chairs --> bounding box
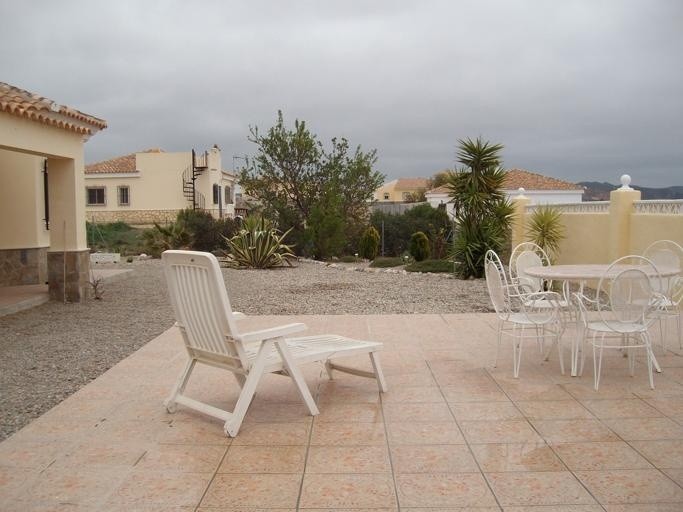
[481,240,682,393]
[157,249,386,441]
